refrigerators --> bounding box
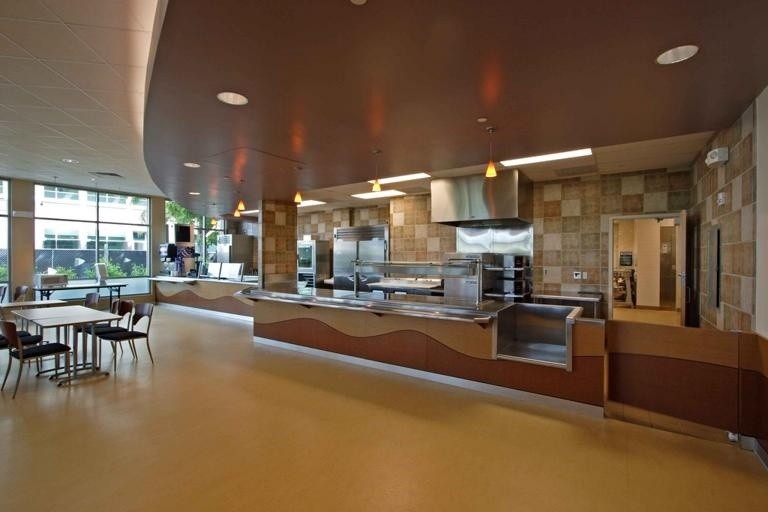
[333,227,389,292]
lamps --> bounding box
[238,180,245,211]
[234,191,240,217]
[485,126,497,178]
[293,167,303,202]
[211,203,216,224]
[372,150,382,192]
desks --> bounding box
[31,283,128,312]
[531,291,602,318]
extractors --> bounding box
[430,169,534,227]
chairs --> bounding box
[0,286,153,399]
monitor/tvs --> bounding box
[175,224,191,242]
[619,250,633,266]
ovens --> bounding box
[296,239,331,289]
